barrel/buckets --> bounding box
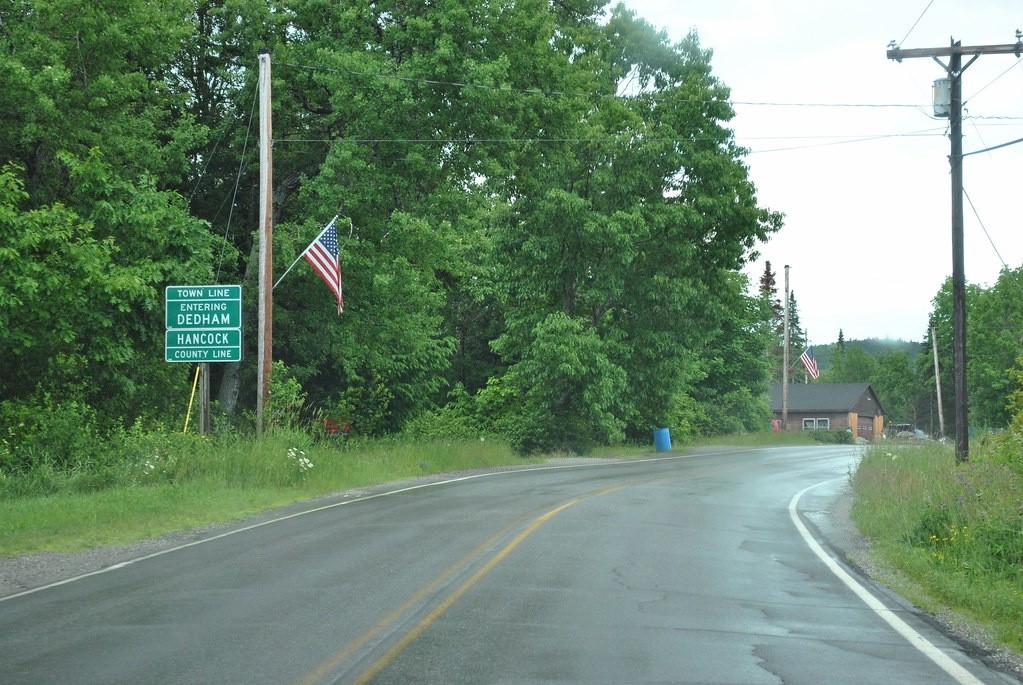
[653,427,671,452]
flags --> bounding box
[303,221,343,315]
[800,346,820,379]
